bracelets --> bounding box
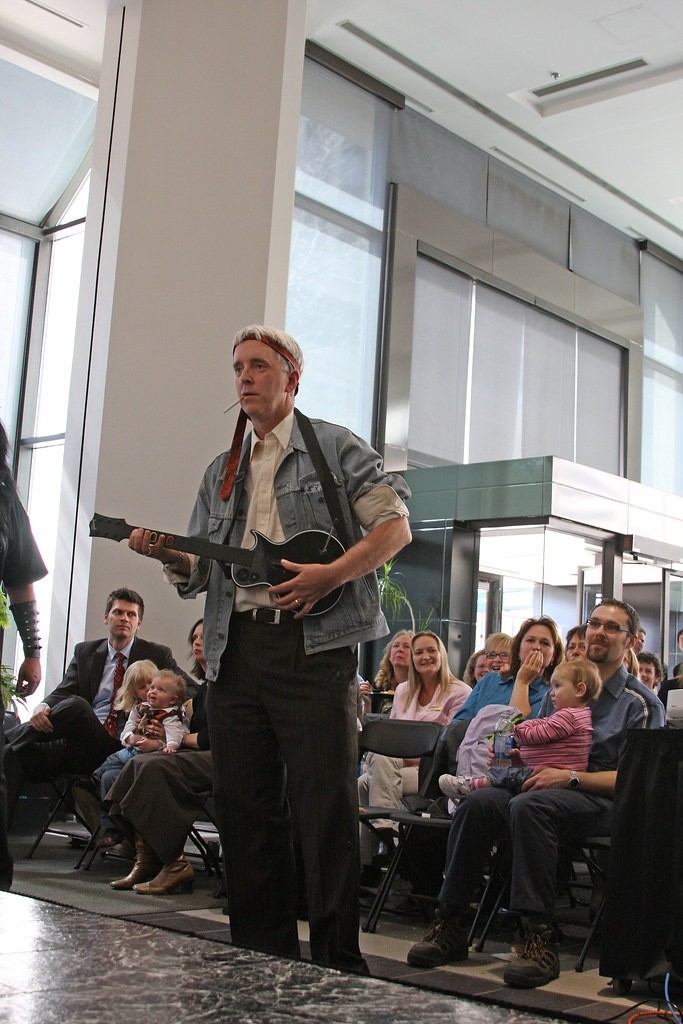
[569,771,580,789]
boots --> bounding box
[109,830,161,889]
[133,854,194,894]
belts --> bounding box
[231,608,301,625]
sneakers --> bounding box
[407,908,468,965]
[503,921,561,988]
[439,774,474,799]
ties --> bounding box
[102,651,127,737]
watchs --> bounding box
[162,551,184,570]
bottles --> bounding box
[492,710,514,767]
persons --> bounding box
[451,615,683,721]
[0,423,49,892]
[73,618,213,894]
[3,588,201,832]
[407,598,666,988]
[357,630,472,888]
[438,659,602,798]
[129,324,413,976]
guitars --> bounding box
[87,512,350,616]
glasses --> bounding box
[586,620,631,634]
[485,652,509,659]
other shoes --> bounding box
[359,865,383,887]
[111,837,130,857]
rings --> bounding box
[295,599,300,606]
[145,547,151,556]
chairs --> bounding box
[357,717,442,930]
[368,718,542,946]
[473,831,616,971]
[21,772,229,899]
